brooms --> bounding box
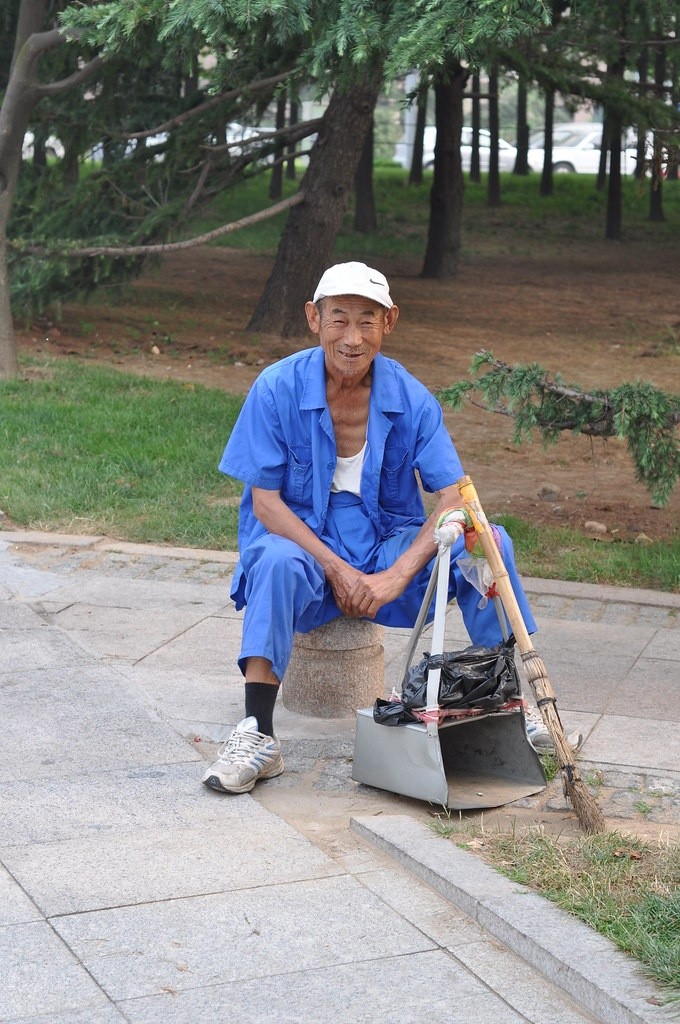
[457,474,607,837]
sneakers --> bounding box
[525,711,583,756]
[202,716,285,794]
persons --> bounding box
[202,261,583,797]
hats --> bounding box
[313,261,394,310]
